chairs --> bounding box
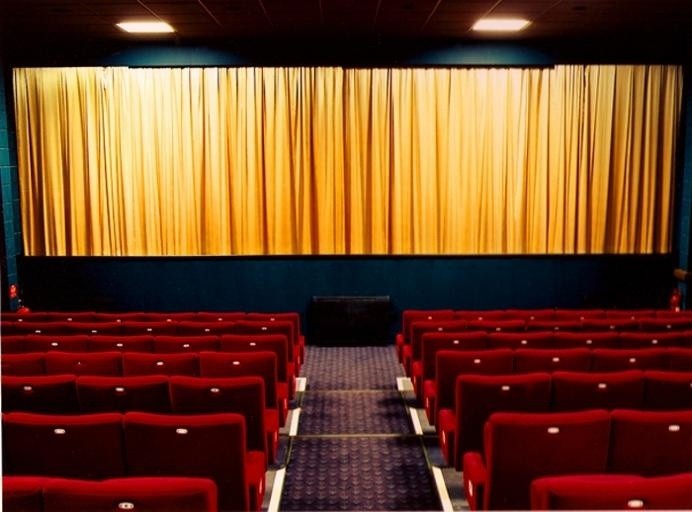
[393,303,692,510]
[0,308,313,512]
